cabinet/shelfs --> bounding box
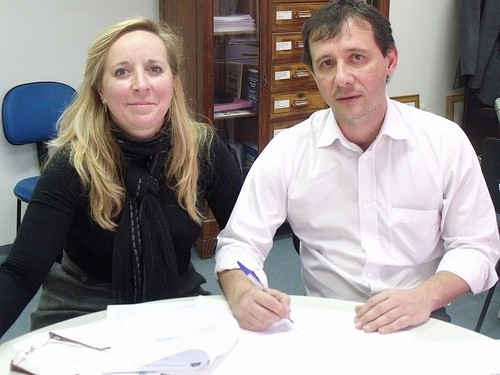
[159,0,391,259]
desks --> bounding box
[0,294,500,375]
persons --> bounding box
[214,0,500,333]
[0,17,243,337]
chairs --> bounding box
[2,81,79,231]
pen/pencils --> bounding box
[236,260,295,324]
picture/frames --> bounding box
[446,94,464,127]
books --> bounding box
[215,62,258,116]
[227,140,258,179]
